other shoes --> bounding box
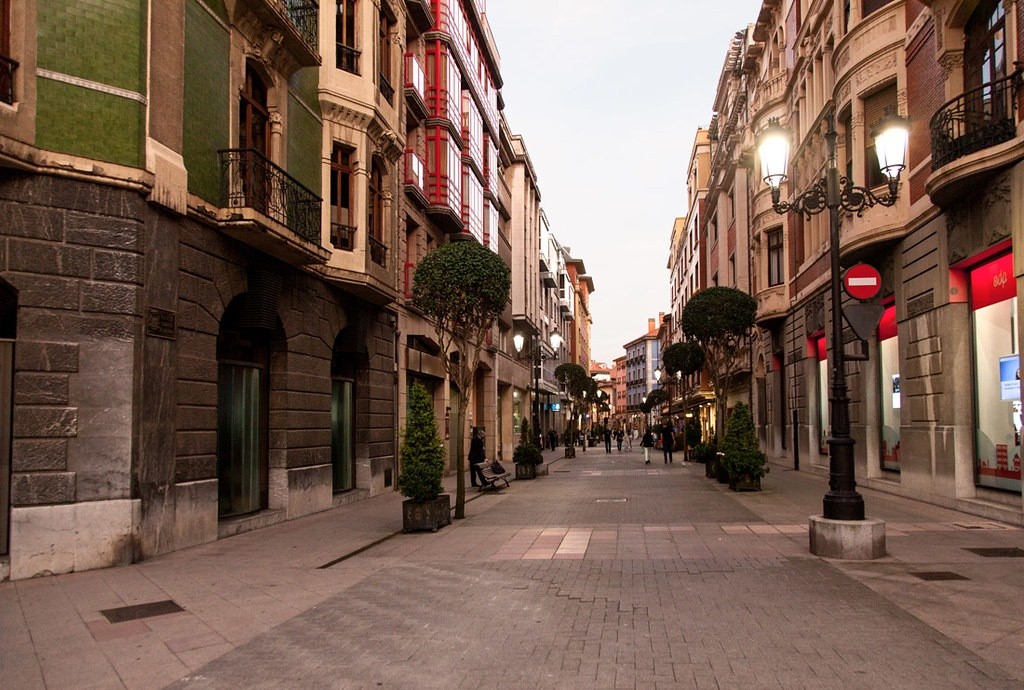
[484,482,490,487]
[472,483,480,487]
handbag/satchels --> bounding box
[491,460,505,475]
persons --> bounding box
[547,426,634,454]
[640,421,675,465]
[467,430,490,487]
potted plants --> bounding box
[720,399,766,490]
[703,428,720,477]
[399,378,450,531]
[685,415,702,462]
[513,415,543,480]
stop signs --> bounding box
[843,264,882,302]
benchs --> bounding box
[474,460,512,492]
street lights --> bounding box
[757,109,913,559]
[514,327,562,450]
[654,365,682,422]
